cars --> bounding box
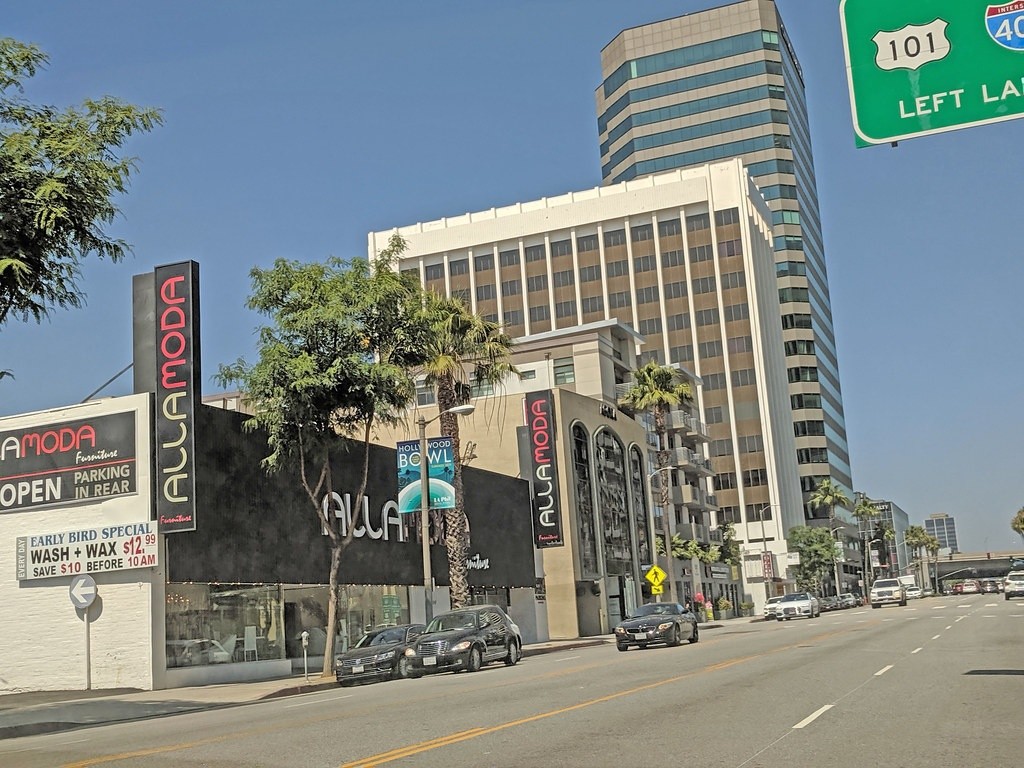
[404,604,521,676]
[335,623,428,685]
[945,564,1024,601]
[764,590,865,623]
[904,586,924,600]
[614,601,698,652]
[923,588,935,597]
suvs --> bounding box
[869,578,908,609]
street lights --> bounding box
[417,402,478,628]
[646,465,679,603]
[830,524,846,596]
[759,504,781,598]
[867,539,883,583]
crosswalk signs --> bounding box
[645,565,669,587]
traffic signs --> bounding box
[836,0,1024,158]
